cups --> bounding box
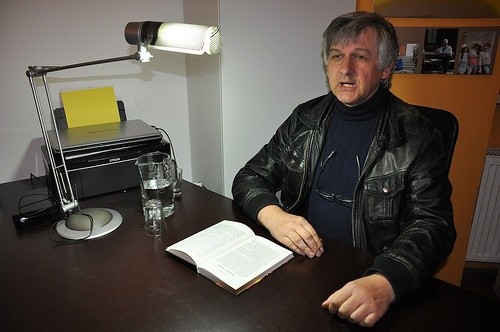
[173,168,182,198]
[143,198,168,236]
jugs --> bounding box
[135,151,176,219]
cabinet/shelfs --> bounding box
[355,0,500,287]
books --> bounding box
[164,219,293,296]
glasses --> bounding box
[316,151,361,207]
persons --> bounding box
[231,10,460,326]
[435,39,454,74]
[457,39,492,74]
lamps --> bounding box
[26,21,222,239]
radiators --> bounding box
[464,151,500,263]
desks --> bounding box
[1,173,500,332]
